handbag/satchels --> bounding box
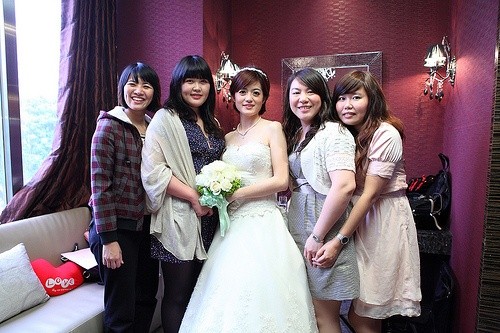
[60,242,100,284]
[404,192,443,231]
[426,152,451,230]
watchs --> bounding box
[337,233,349,244]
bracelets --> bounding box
[311,234,324,243]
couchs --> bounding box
[0,207,105,333]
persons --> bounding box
[178,67,319,333]
[89,62,163,333]
[141,55,225,333]
[312,70,423,333]
[280,68,360,333]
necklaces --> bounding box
[237,117,261,136]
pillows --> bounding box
[31,257,85,296]
[0,242,51,322]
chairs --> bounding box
[406,152,450,230]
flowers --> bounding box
[195,160,241,197]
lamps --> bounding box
[423,32,456,102]
[215,51,240,110]
